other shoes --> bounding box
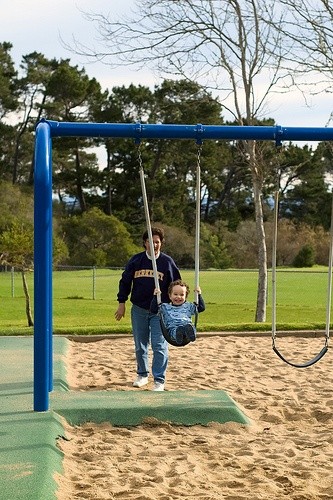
[176,325,183,344]
[132,375,148,388]
[183,323,195,342]
[150,381,164,392]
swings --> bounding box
[271,148,333,368]
[137,146,203,347]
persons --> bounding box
[150,279,205,347]
[115,228,182,391]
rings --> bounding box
[116,314,117,315]
